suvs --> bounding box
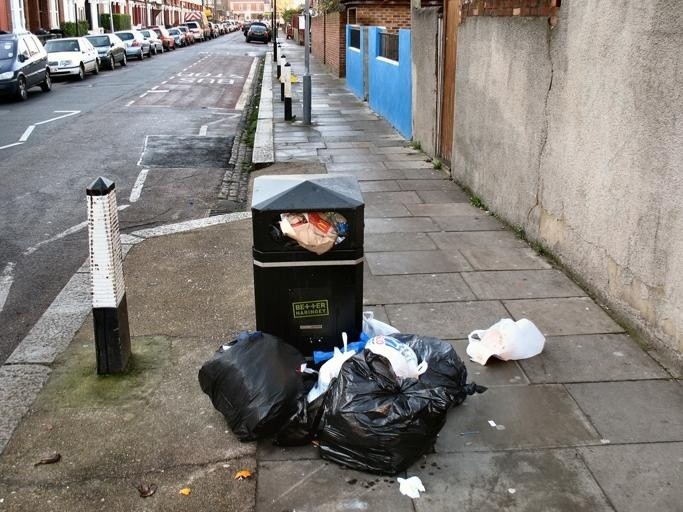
[0,31,52,101]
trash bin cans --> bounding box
[250,172,366,362]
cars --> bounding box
[83,33,128,70]
[113,19,271,71]
[42,36,101,81]
